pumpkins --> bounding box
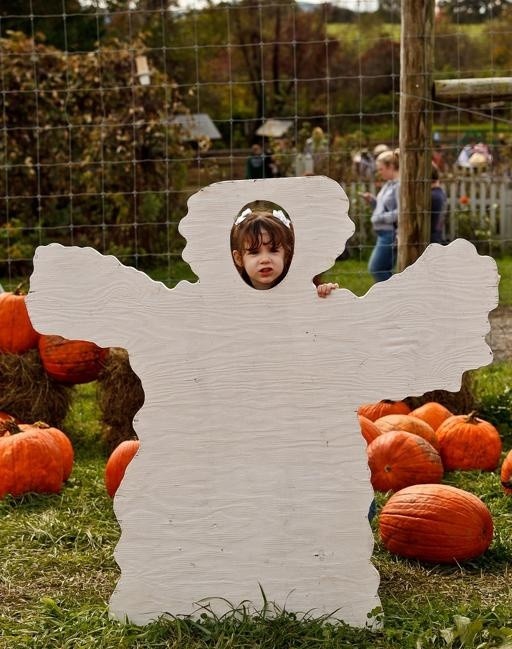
[358,401,512,563]
[0,279,139,503]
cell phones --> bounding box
[357,192,364,197]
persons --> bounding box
[357,150,401,288]
[429,162,448,246]
[247,139,316,178]
[229,206,344,300]
